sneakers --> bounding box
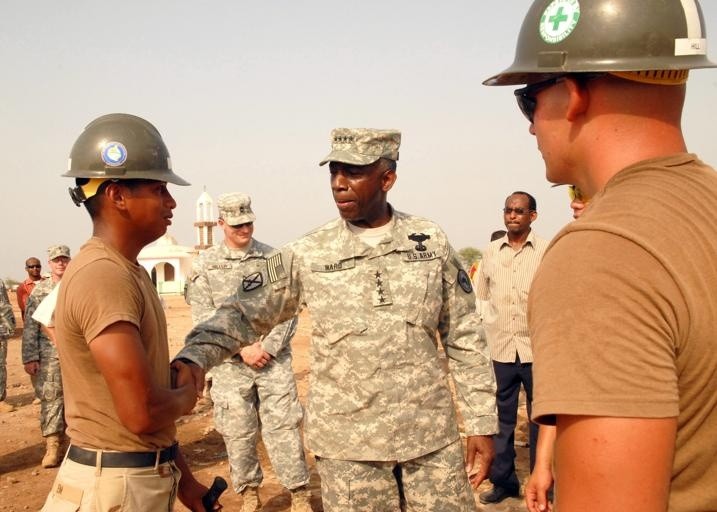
[479,486,519,504]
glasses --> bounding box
[504,207,531,214]
[514,73,590,124]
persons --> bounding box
[477,0,717,512]
[181,193,313,512]
[0,278,18,403]
[38,112,229,512]
[476,191,557,504]
[21,244,72,470]
[169,123,500,512]
[522,181,589,512]
[18,257,48,407]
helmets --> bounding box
[481,0,717,85]
[59,114,190,186]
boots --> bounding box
[42,432,70,468]
[290,487,312,512]
[239,488,263,512]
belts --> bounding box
[67,440,178,467]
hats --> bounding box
[48,245,70,260]
[219,193,255,226]
[319,127,400,166]
